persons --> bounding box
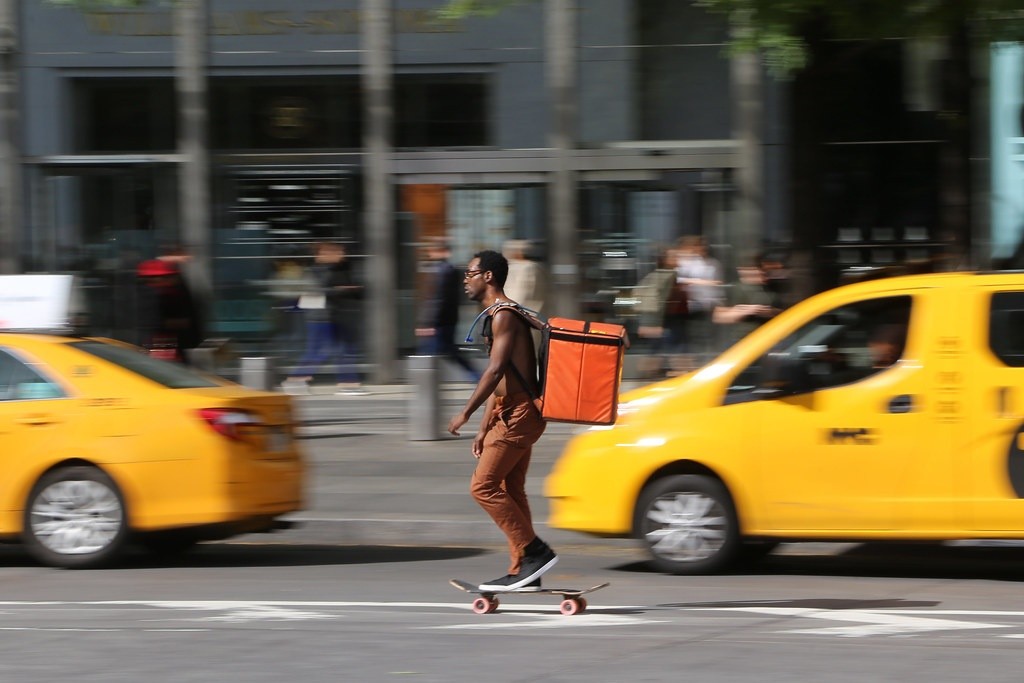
[449,250,560,596]
[255,232,904,395]
[133,242,193,365]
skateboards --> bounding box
[448,577,611,616]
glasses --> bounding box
[463,269,487,278]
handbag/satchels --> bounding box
[297,292,326,309]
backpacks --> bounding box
[481,306,631,426]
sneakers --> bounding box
[479,574,541,594]
[507,536,560,589]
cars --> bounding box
[0,334,308,569]
[542,270,1023,576]
